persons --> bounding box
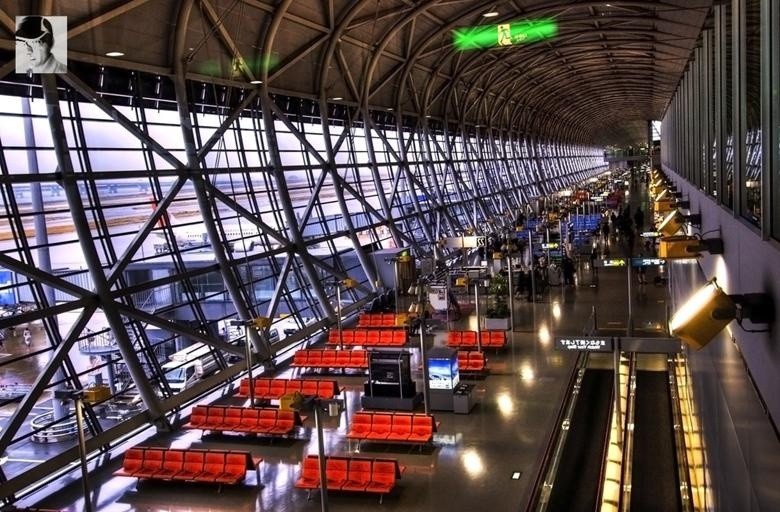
[15,16,67,73]
[23,327,31,348]
[635,253,647,283]
[515,248,598,299]
[603,204,644,242]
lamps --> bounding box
[644,160,725,263]
[667,277,770,353]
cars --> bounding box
[283,318,316,336]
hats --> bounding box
[15,17,52,42]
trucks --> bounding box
[159,342,230,402]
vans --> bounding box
[229,328,280,361]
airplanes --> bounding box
[139,197,291,253]
